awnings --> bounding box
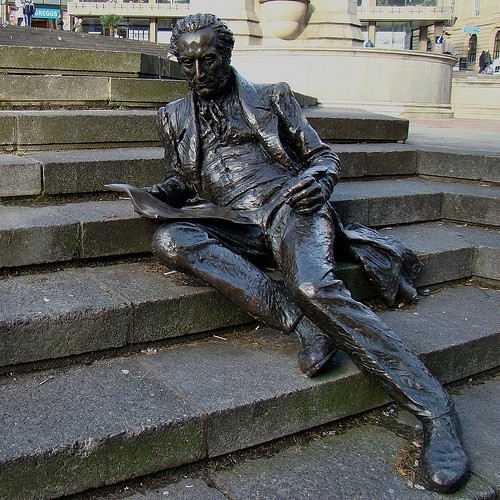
[463,27,480,34]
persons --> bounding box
[16,0,84,33]
[133,13,473,492]
[478,49,492,73]
[365,39,374,47]
